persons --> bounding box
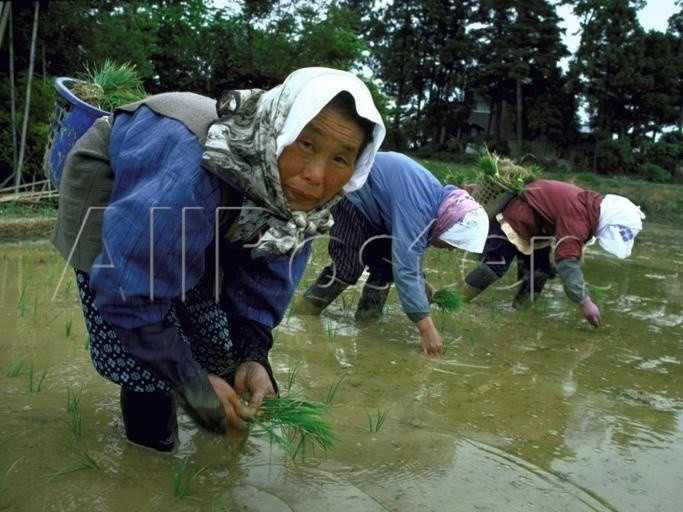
[451,179,643,328]
[288,150,490,358]
[49,67,387,454]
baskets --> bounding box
[471,172,514,217]
[42,76,111,189]
